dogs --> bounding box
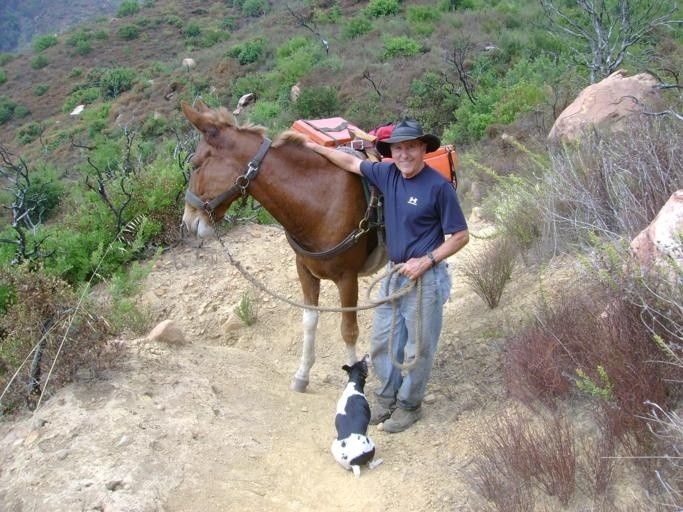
[330,353,383,479]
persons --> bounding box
[280,120,469,432]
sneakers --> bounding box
[368,405,396,425]
[377,410,421,431]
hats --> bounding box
[376,116,440,157]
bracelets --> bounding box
[425,250,435,268]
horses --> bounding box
[180,96,389,393]
